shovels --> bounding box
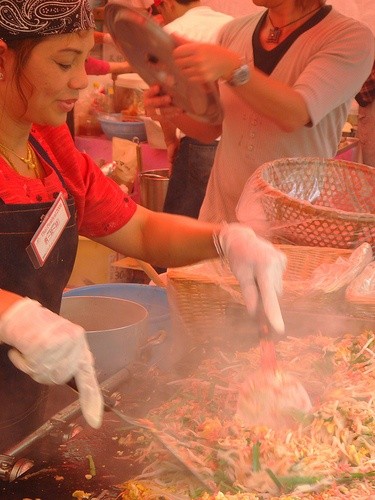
[236,278,312,430]
[65,376,219,493]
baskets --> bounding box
[167,246,375,342]
[257,159,375,247]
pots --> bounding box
[58,296,166,377]
[137,169,170,212]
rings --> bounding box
[155,108,161,115]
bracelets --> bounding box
[227,63,250,85]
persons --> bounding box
[143,0,375,224]
[354,61,375,170]
[153,0,237,274]
[0,0,287,456]
[66,30,139,141]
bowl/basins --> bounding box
[96,113,146,141]
[63,281,172,345]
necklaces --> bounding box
[266,6,321,43]
[0,144,40,181]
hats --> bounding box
[0,0,97,40]
